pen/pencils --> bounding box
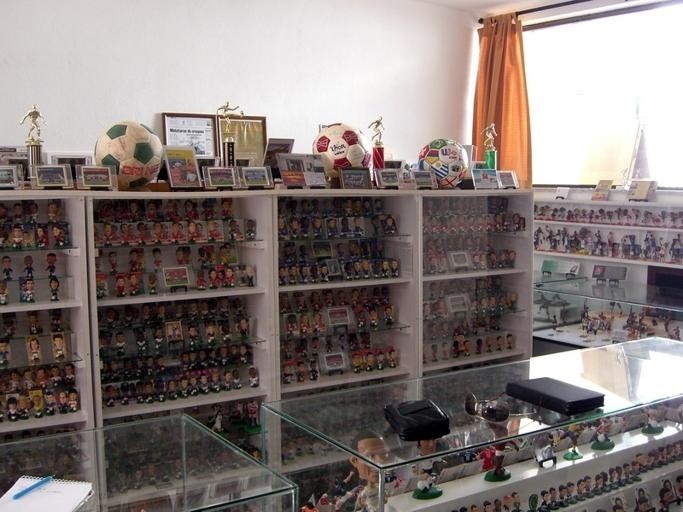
[13,474,56,500]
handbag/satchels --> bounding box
[383,398,450,442]
[506,377,605,415]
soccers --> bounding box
[94,121,163,190]
[418,139,469,189]
[312,122,371,179]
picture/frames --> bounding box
[163,112,267,160]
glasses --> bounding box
[463,392,543,424]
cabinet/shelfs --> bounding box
[0,189,535,512]
[380,396,683,512]
[535,199,682,352]
